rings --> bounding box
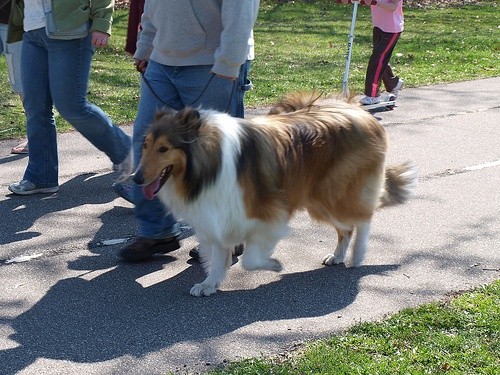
[102,39,106,41]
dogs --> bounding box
[132,87,416,297]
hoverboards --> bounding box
[336,0,397,112]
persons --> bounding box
[335,0,404,105]
[0,0,260,259]
[117,0,261,259]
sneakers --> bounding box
[190,244,244,257]
[8,179,59,195]
[359,96,381,104]
[391,79,404,96]
[119,237,180,259]
[111,150,132,187]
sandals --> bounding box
[11,142,28,154]
